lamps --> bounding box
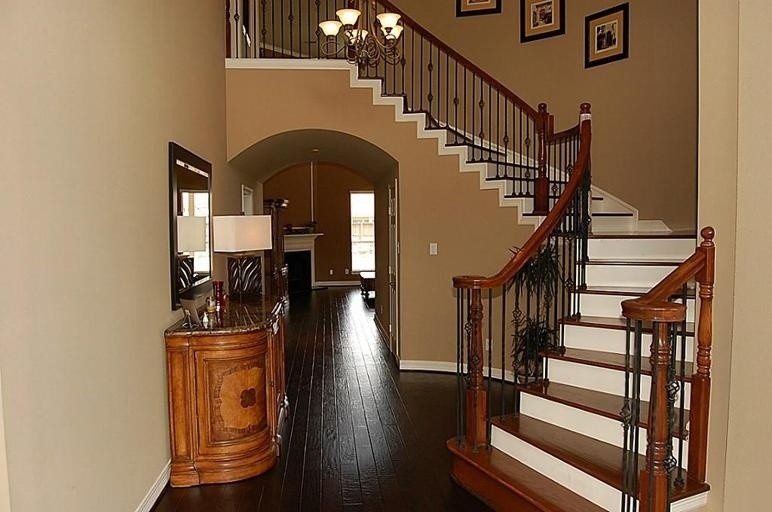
[176,215,206,252]
[315,0,405,69]
[211,213,274,307]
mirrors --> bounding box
[168,141,213,311]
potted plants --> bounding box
[502,242,567,389]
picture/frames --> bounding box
[519,0,567,45]
[582,1,631,72]
[454,0,504,19]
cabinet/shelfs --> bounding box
[163,297,291,489]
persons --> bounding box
[533,2,551,27]
[597,29,616,49]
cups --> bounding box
[206,300,217,313]
